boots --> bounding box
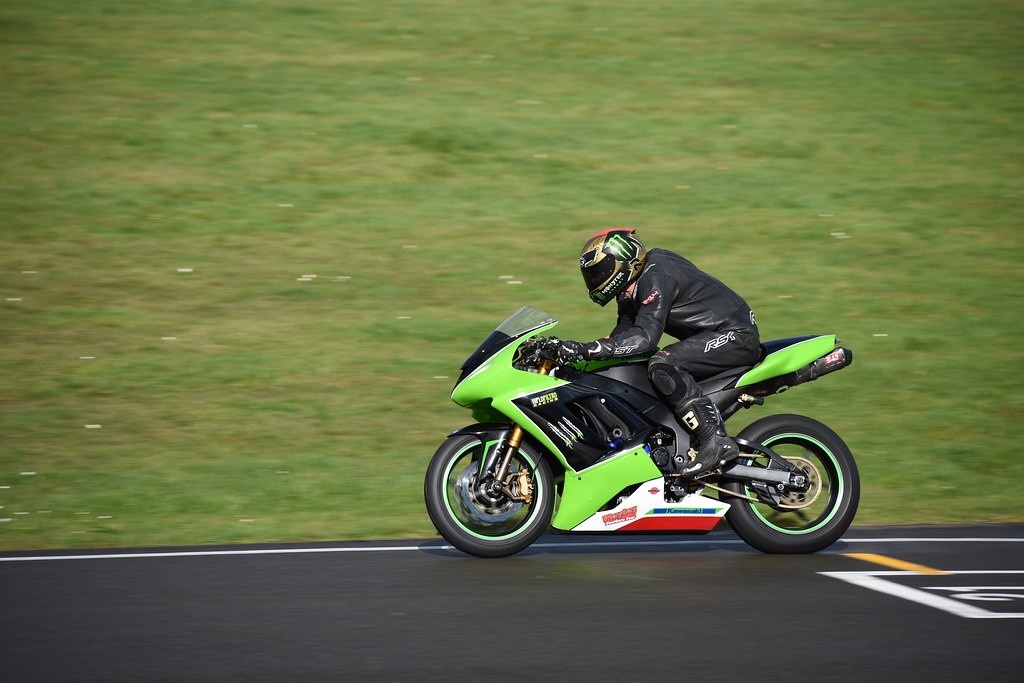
[678,395,740,477]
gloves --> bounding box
[558,337,591,364]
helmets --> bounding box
[580,227,646,307]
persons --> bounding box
[521,228,760,477]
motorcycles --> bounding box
[424,305,861,559]
